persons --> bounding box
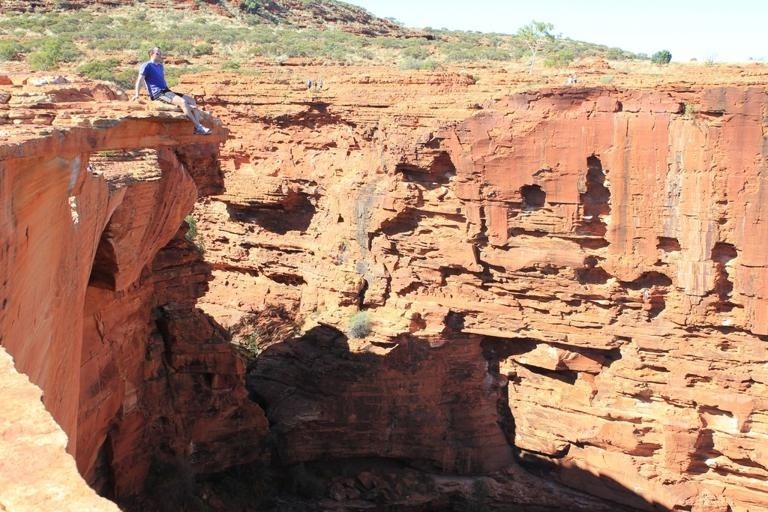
[133,47,212,134]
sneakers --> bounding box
[193,124,211,134]
